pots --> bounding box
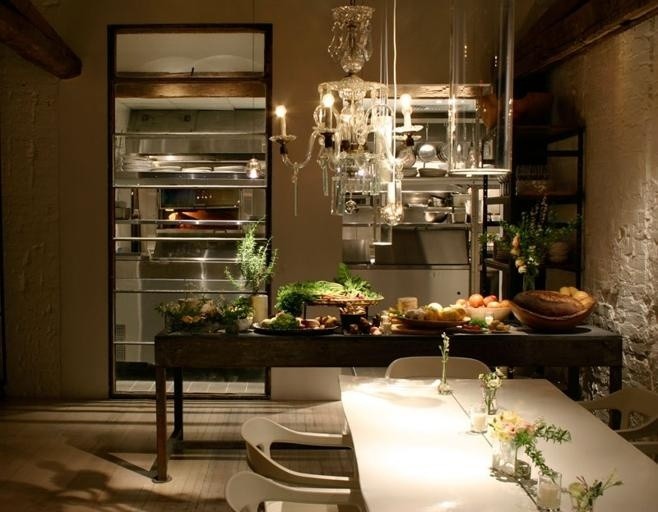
[415,125,448,163]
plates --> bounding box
[395,316,471,327]
[123,155,245,174]
[253,323,339,334]
[403,168,447,177]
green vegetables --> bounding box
[275,279,347,316]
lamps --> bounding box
[267,0,425,227]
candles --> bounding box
[538,472,562,508]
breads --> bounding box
[514,286,594,316]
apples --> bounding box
[457,294,512,309]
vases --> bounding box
[478,391,500,415]
[490,438,517,476]
[523,274,535,289]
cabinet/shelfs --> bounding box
[480,122,585,320]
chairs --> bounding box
[576,382,658,440]
[627,441,657,463]
[239,417,351,489]
[384,356,496,379]
[225,470,351,512]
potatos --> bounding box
[261,312,338,328]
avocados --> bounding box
[347,316,370,334]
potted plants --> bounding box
[221,213,280,332]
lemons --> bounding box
[426,302,466,321]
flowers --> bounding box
[487,410,534,440]
[474,367,509,389]
[569,467,619,511]
[477,202,582,290]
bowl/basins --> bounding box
[423,210,448,223]
[451,302,597,333]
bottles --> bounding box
[246,158,261,178]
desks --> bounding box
[154,322,622,483]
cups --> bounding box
[382,317,392,336]
[484,312,495,325]
[537,469,561,512]
[471,405,488,433]
